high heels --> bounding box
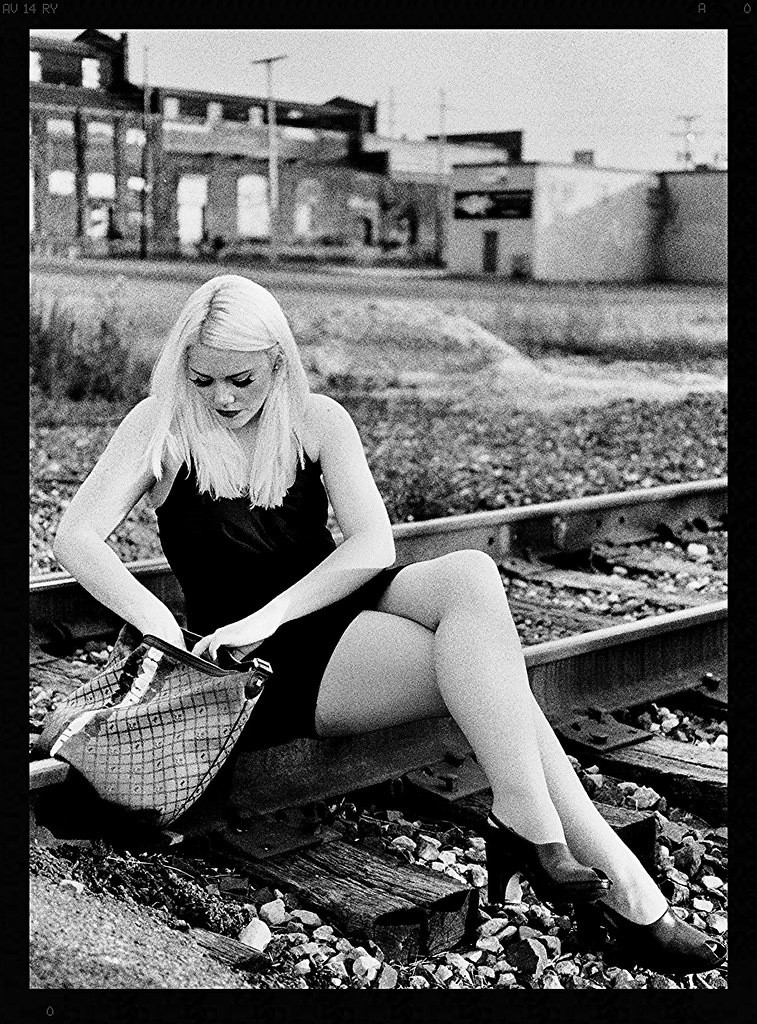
[480,808,614,908]
[574,892,729,975]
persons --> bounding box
[51,275,726,974]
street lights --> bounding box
[252,52,289,267]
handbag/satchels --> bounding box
[31,610,276,841]
